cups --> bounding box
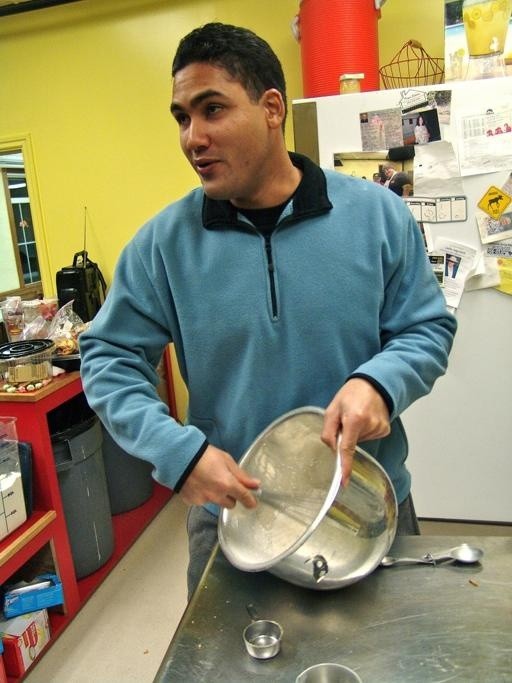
[242,602,283,660]
[377,546,485,569]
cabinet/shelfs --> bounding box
[0,343,179,683]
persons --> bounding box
[78,20,459,608]
[414,114,431,146]
[446,255,460,279]
[487,215,512,235]
[382,161,412,197]
[372,172,385,186]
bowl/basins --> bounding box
[217,405,399,591]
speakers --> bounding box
[56,262,101,323]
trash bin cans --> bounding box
[50,408,156,578]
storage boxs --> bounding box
[0,606,50,678]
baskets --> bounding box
[379,40,444,89]
